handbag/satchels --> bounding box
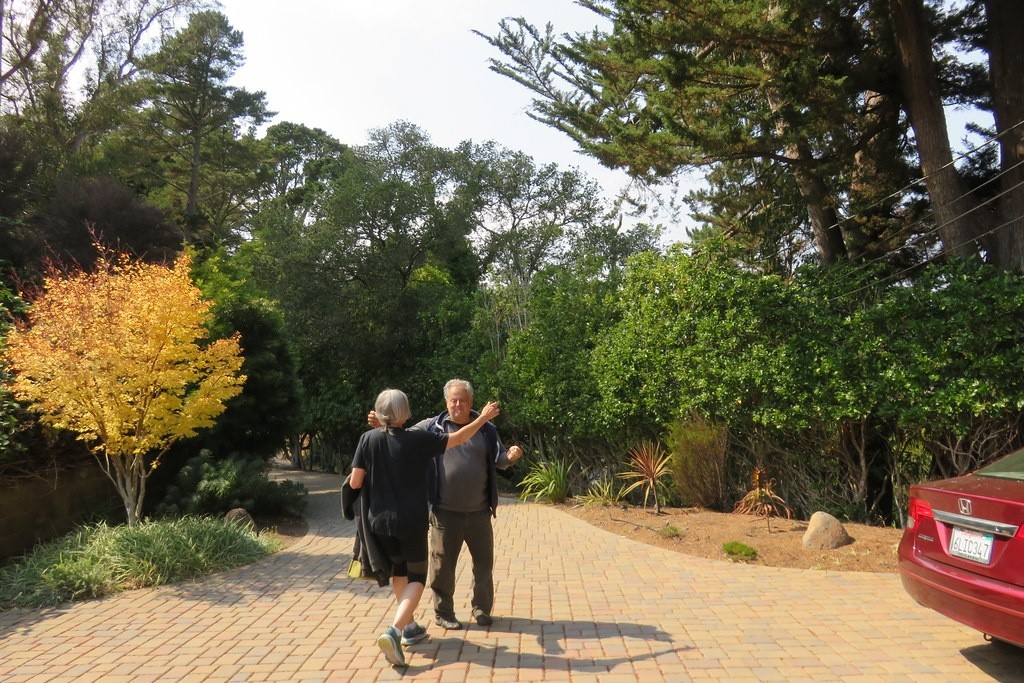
[347,559,376,582]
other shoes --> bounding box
[474,609,492,625]
[436,616,463,629]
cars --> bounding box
[898,446,1024,655]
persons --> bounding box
[346,388,500,668]
[368,378,523,631]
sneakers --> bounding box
[400,622,427,645]
[378,624,405,666]
